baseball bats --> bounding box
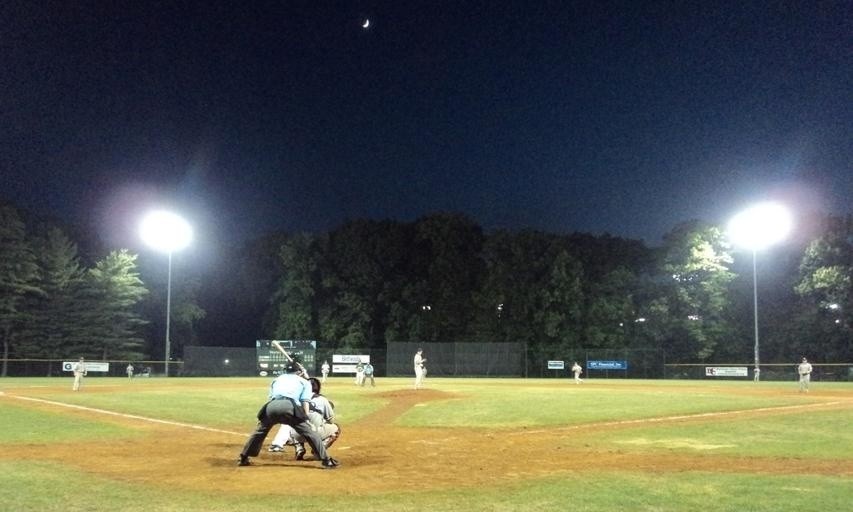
[272,340,293,363]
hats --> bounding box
[284,362,301,372]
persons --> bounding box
[413,348,427,389]
[753,366,760,380]
[571,362,582,384]
[356,361,365,384]
[798,357,813,393]
[72,357,87,390]
[127,363,134,380]
[362,362,374,386]
[237,356,340,467]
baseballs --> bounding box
[225,359,230,363]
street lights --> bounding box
[136,210,192,373]
[734,202,790,380]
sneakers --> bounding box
[294,443,306,461]
[268,445,285,453]
[322,457,342,468]
[237,453,250,467]
